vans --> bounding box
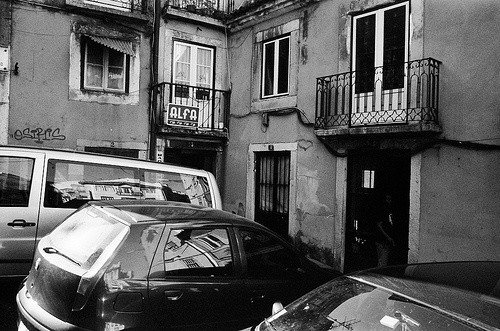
[0,144,224,279]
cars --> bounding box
[15,198,344,331]
[237,259,500,331]
